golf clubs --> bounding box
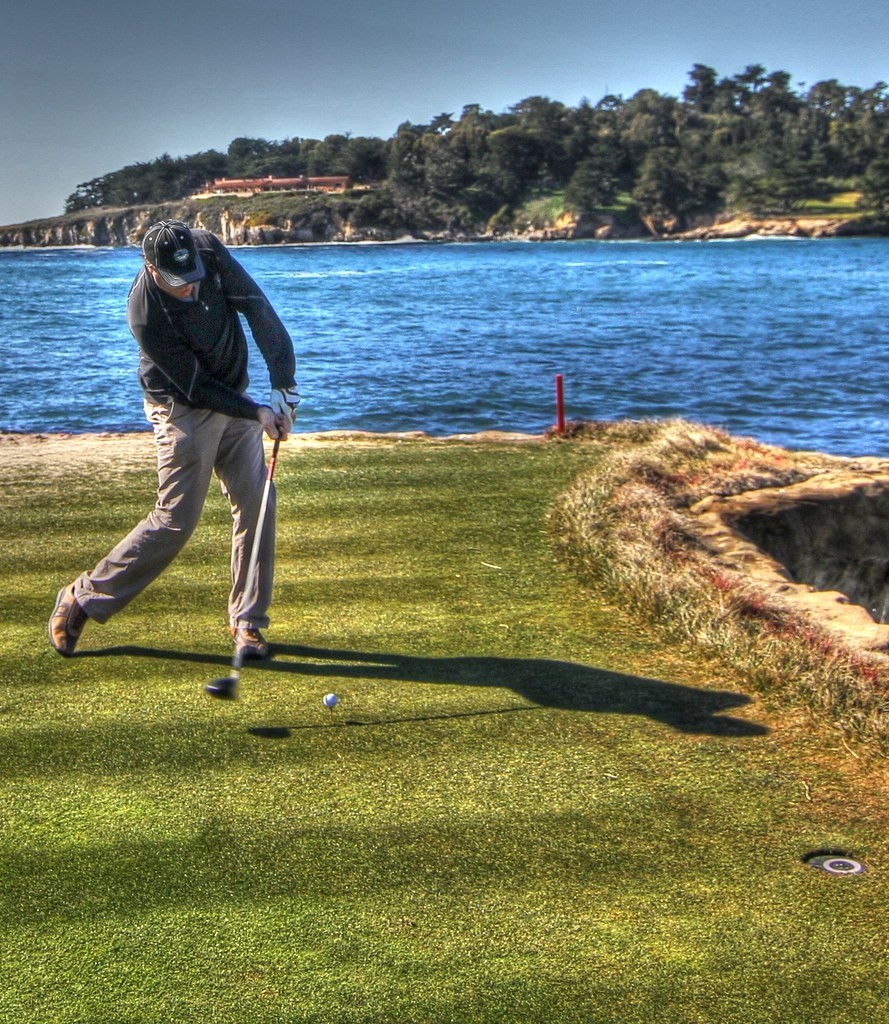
[205,397,286,702]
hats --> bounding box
[142,219,204,288]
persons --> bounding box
[48,220,299,659]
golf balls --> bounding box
[319,690,341,710]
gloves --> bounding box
[271,388,300,424]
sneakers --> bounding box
[229,626,268,661]
[48,580,85,659]
